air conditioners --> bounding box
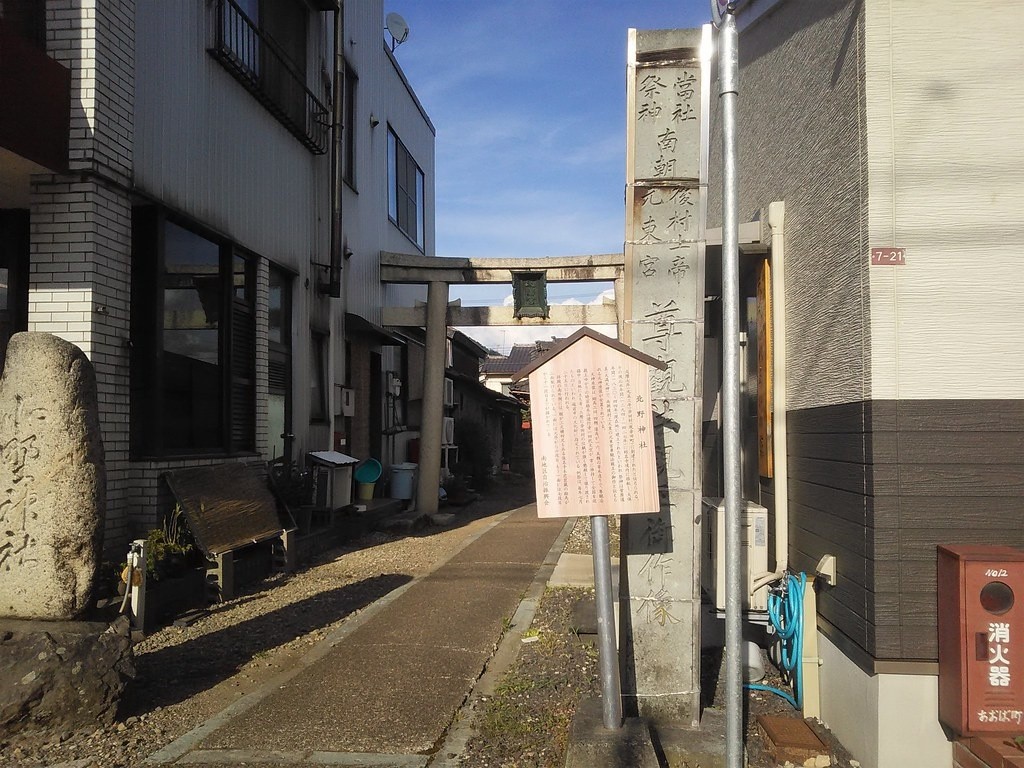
[701,497,768,614]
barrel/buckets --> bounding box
[389,469,417,499]
[357,482,376,501]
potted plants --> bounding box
[271,461,319,533]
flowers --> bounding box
[95,529,185,597]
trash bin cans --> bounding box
[388,462,416,500]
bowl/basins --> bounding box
[353,457,382,483]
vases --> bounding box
[98,566,204,618]
